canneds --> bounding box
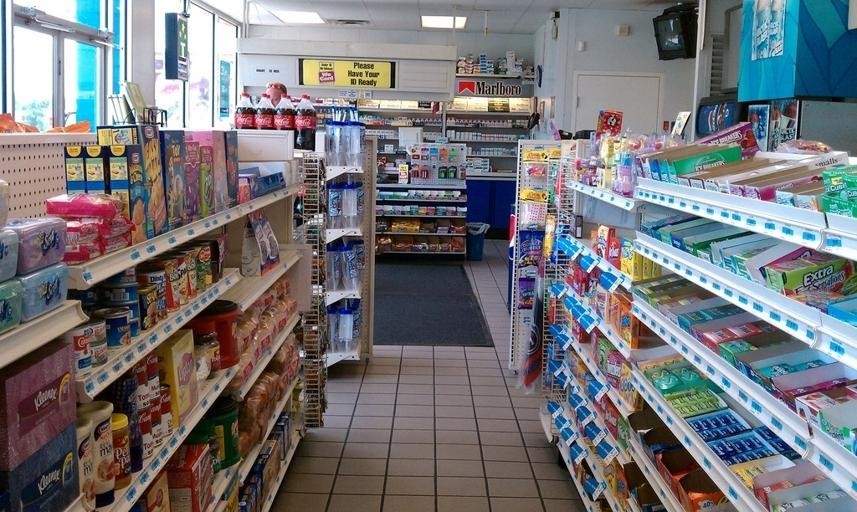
[59,240,221,493]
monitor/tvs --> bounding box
[718,94,769,132]
[694,96,723,138]
[652,2,699,61]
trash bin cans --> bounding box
[466,222,484,261]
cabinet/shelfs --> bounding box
[375,141,473,260]
[506,135,565,397]
[358,98,538,184]
[0,112,332,512]
[537,133,857,512]
[735,0,857,105]
[466,176,519,240]
[320,128,378,377]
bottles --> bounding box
[359,114,442,127]
[321,120,367,356]
[234,92,315,152]
[446,116,531,156]
